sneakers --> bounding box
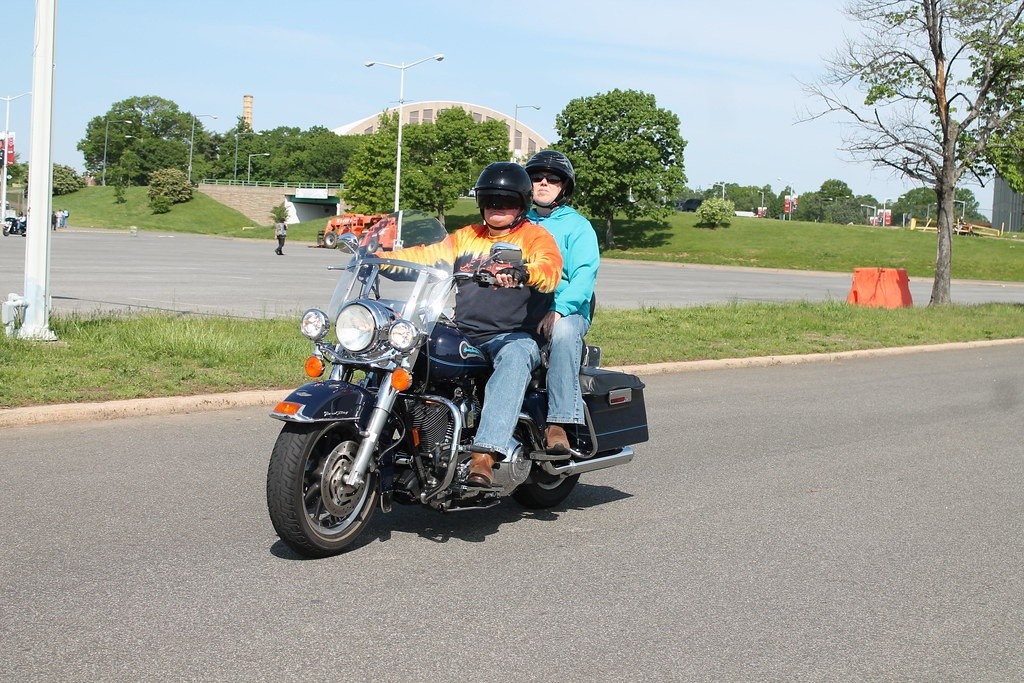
[544,425,570,454]
[466,452,495,488]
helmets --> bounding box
[475,162,533,208]
[526,150,575,197]
[18,211,23,216]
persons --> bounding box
[523,151,600,455]
[16,211,26,233]
[351,162,564,490]
[51,208,70,231]
[275,218,288,255]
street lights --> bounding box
[234,132,265,182]
[247,152,273,185]
[364,55,447,214]
[187,114,225,186]
[777,178,794,222]
[1,92,34,228]
[882,195,906,228]
[758,190,764,218]
[101,118,137,188]
[714,183,726,202]
[860,204,876,227]
[512,104,543,123]
[953,199,968,221]
[927,202,937,221]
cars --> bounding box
[671,197,702,212]
[364,213,448,246]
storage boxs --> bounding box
[580,366,649,452]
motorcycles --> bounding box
[2,216,28,237]
[263,209,638,553]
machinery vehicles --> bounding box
[314,211,392,251]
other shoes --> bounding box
[275,249,279,255]
[278,253,284,255]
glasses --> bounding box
[530,172,562,184]
[477,189,522,208]
